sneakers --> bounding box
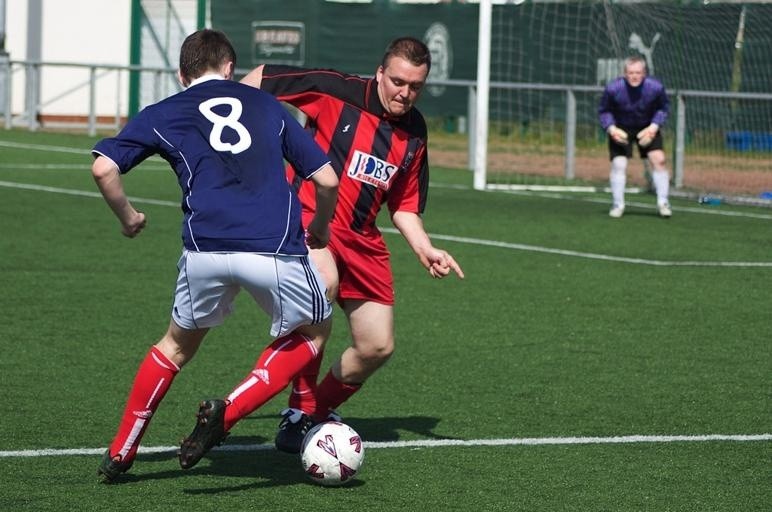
[658,203,672,217]
[97,449,134,484]
[275,407,342,455]
[176,399,231,469]
[609,205,625,218]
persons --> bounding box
[237,37,465,453]
[91,29,339,485]
[597,52,672,219]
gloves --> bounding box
[607,124,629,146]
[637,122,659,147]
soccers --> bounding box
[299,421,364,488]
